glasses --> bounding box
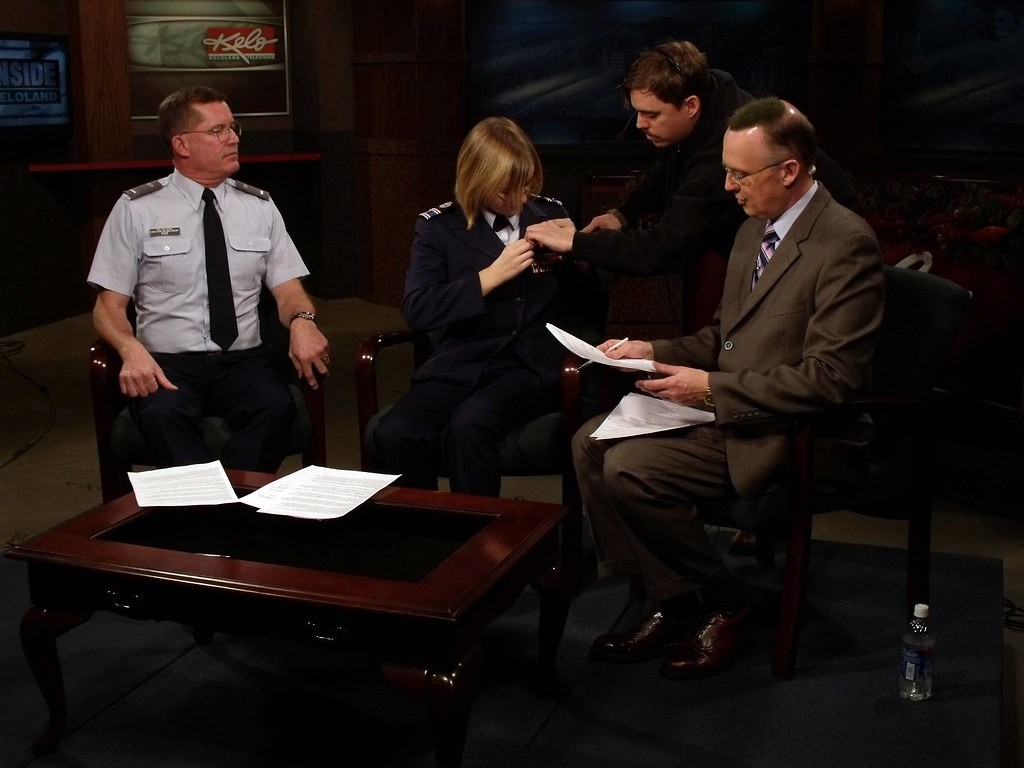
[177,120,243,144]
[495,171,542,205]
[719,155,793,182]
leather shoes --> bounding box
[665,585,756,673]
[590,562,697,680]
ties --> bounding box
[751,224,781,291]
[201,188,239,350]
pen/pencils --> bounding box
[575,337,629,372]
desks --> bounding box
[0,468,570,768]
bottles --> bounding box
[897,604,937,702]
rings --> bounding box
[321,352,329,361]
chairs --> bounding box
[87,282,328,503]
[628,261,974,683]
[354,257,611,599]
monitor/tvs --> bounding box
[0,32,74,140]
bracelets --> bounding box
[607,208,628,228]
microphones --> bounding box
[616,111,637,143]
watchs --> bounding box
[289,311,318,326]
[703,385,715,413]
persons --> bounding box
[87,85,332,498]
[571,98,885,672]
[526,40,864,277]
[373,117,576,499]
